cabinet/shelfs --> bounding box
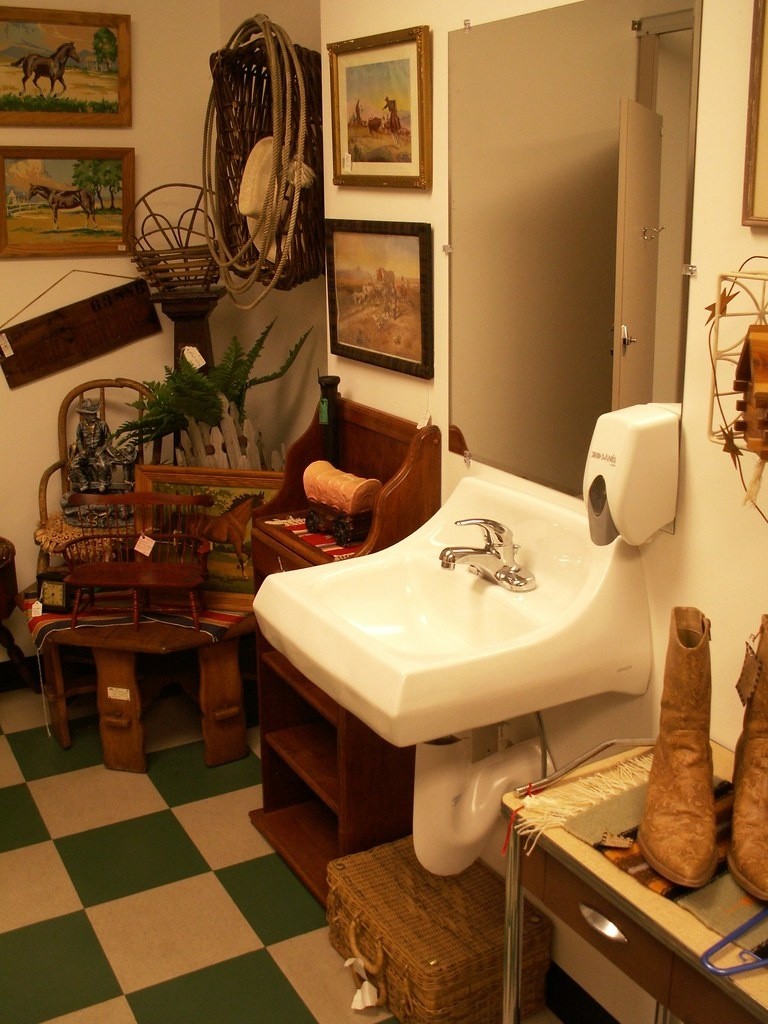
[247,393,444,909]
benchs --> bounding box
[53,532,212,631]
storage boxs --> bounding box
[40,581,68,614]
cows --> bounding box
[368,116,386,137]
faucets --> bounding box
[438,518,537,591]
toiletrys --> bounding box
[583,401,679,546]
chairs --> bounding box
[64,485,217,668]
[33,376,164,585]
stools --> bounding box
[0,536,42,704]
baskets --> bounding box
[125,184,224,296]
[210,35,326,290]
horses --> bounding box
[11,42,80,96]
[26,183,98,232]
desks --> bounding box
[22,590,256,776]
[501,737,768,1024]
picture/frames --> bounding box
[0,5,132,126]
[322,217,436,381]
[132,460,287,611]
[737,0,768,229]
[325,23,434,193]
[0,144,138,260]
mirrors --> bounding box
[446,0,704,537]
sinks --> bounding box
[250,467,653,747]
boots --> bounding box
[727,614,768,902]
[637,607,718,888]
